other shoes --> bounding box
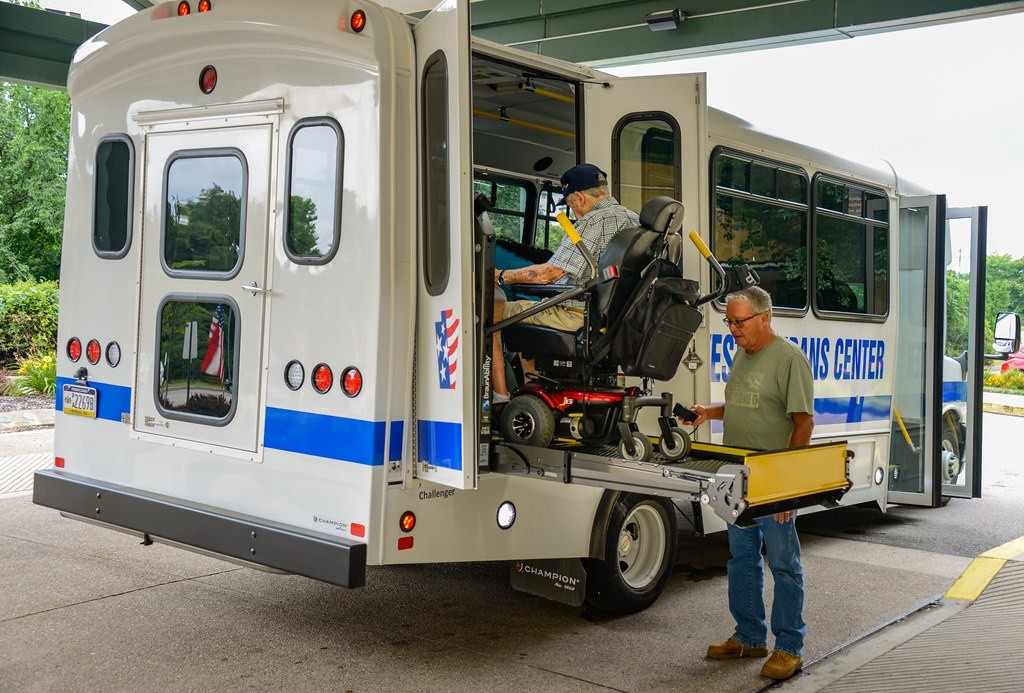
[490,402,512,435]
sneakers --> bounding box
[761,650,804,679]
[707,635,768,660]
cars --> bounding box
[1001,344,1024,374]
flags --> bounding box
[200,306,224,387]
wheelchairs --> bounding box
[491,190,729,463]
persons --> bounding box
[491,164,641,414]
[676,285,814,679]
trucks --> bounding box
[26,1,1024,620]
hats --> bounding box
[555,164,607,206]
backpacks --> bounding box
[609,243,703,381]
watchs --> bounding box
[498,270,505,285]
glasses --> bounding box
[723,313,763,329]
